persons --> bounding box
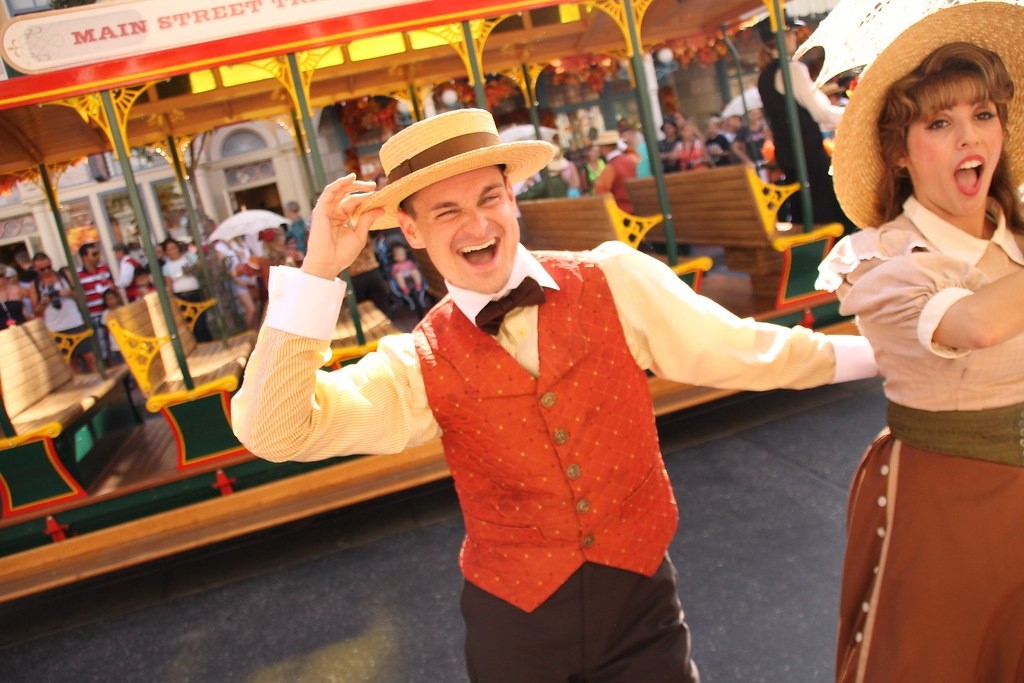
[230,106,882,683]
[0,173,429,372]
[753,9,859,238]
[814,0,1024,683]
[548,107,790,196]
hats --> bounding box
[830,3,1024,232]
[660,116,678,132]
[351,106,561,233]
[758,9,808,41]
[593,129,620,146]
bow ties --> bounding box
[473,275,547,335]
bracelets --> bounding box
[41,301,47,306]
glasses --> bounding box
[91,251,101,257]
[39,266,52,271]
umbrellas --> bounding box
[719,88,765,123]
[206,205,290,254]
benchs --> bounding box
[103,285,258,414]
[516,193,713,275]
[626,162,844,251]
[285,250,404,371]
[0,318,129,448]
